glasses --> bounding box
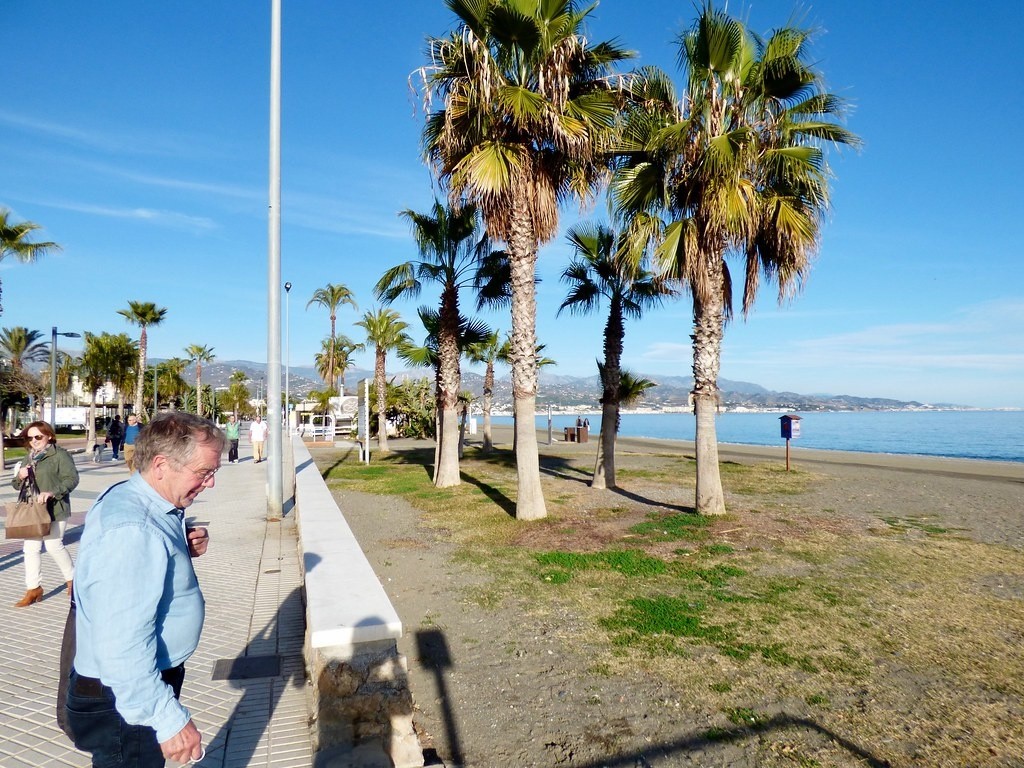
[27,435,45,441]
[176,460,219,481]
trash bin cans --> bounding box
[564,427,575,442]
[577,427,588,443]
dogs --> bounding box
[93,443,108,462]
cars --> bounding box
[242,413,267,422]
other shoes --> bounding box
[229,459,238,464]
[254,460,261,463]
[112,458,117,461]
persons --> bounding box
[225,415,240,464]
[12,420,81,608]
[247,415,268,463]
[105,413,146,476]
[56,410,234,768]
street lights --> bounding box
[51,326,82,432]
[154,364,170,418]
[285,281,292,437]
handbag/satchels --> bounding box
[56,607,76,742]
[5,466,51,539]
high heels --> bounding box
[15,586,43,607]
[66,581,73,598]
